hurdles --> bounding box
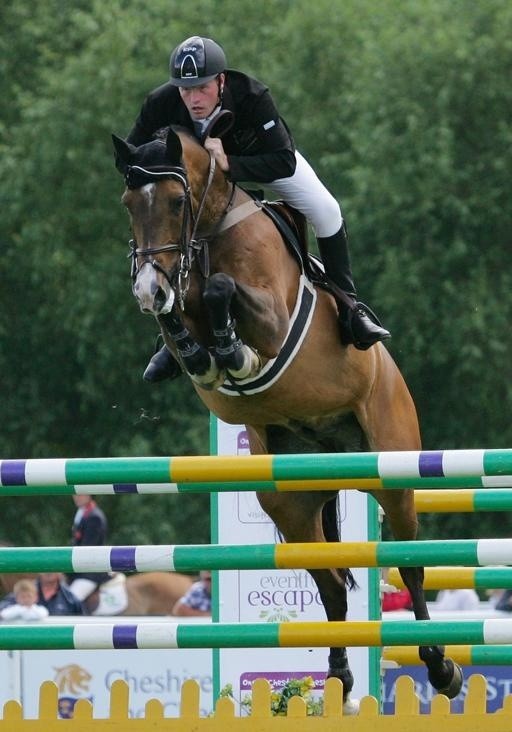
[0,445,512,730]
[209,410,512,715]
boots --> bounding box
[141,342,175,386]
[315,222,392,345]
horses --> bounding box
[0,542,202,618]
[108,125,462,704]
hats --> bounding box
[169,35,226,85]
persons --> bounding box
[481,562,512,613]
[1,572,88,616]
[2,579,50,624]
[68,492,117,610]
[127,31,396,387]
[429,586,479,614]
[174,567,212,618]
